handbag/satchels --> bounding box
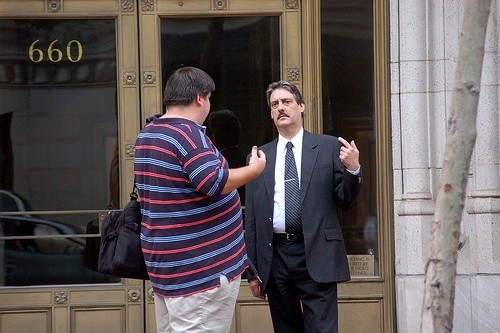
[97,190,150,281]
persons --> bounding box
[133,66,266,333]
[106,149,119,209]
[210,107,247,205]
[245,80,362,333]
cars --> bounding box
[0,214,88,284]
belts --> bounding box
[273,231,303,242]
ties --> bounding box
[283,142,303,234]
